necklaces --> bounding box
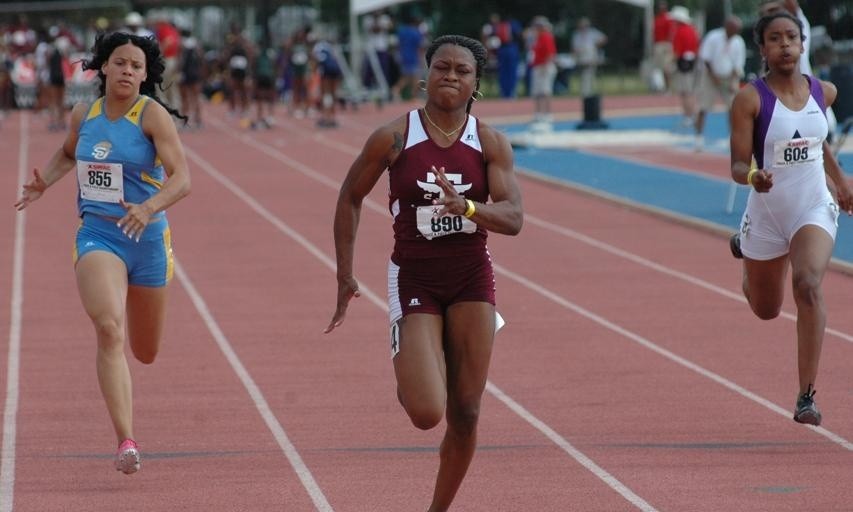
[424,107,468,143]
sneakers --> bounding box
[114,439,140,475]
[793,394,821,425]
[730,233,743,260]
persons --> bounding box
[720,4,851,426]
[11,27,194,476]
[0,0,852,138]
[320,32,528,512]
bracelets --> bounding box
[462,198,476,219]
[746,168,759,186]
